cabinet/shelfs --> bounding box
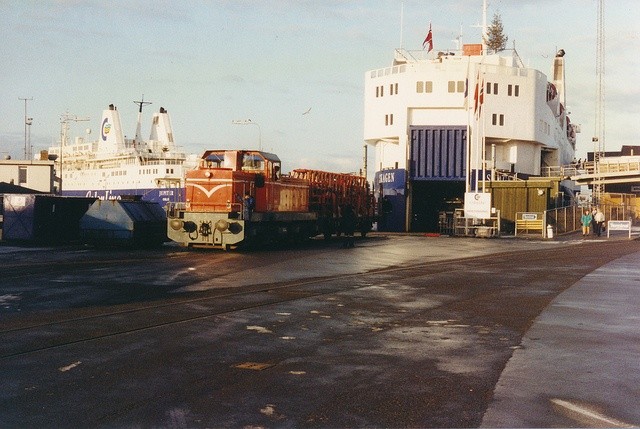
[454,207,500,239]
[439,211,454,235]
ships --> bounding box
[34,94,201,206]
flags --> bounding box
[476,78,484,121]
[464,66,469,108]
[473,72,479,114]
[423,28,433,56]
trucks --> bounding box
[166,150,382,252]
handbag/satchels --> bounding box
[602,225,606,233]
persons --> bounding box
[580,210,591,237]
[594,209,604,237]
[592,207,597,233]
